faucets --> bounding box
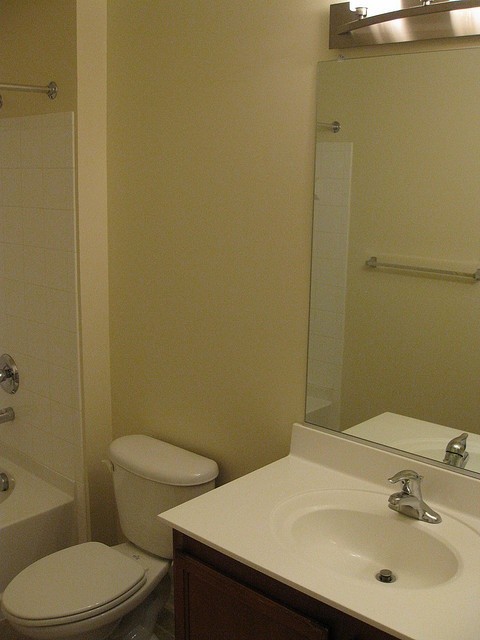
[387,470,442,524]
[443,432,469,469]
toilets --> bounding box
[1,433,220,640]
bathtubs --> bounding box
[1,456,72,529]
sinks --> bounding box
[272,489,479,594]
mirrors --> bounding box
[303,47,480,476]
